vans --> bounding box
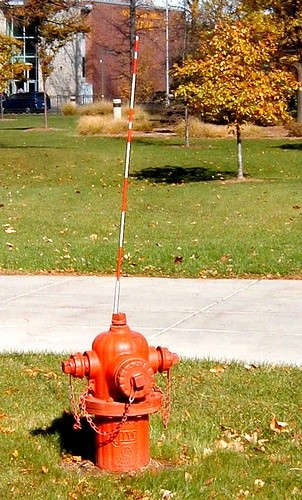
[1,91,51,113]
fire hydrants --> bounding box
[61,310,181,475]
